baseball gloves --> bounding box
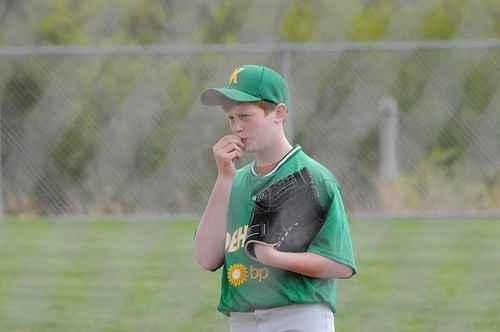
[240,166,329,262]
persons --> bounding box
[192,64,358,332]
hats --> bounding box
[201,64,290,113]
